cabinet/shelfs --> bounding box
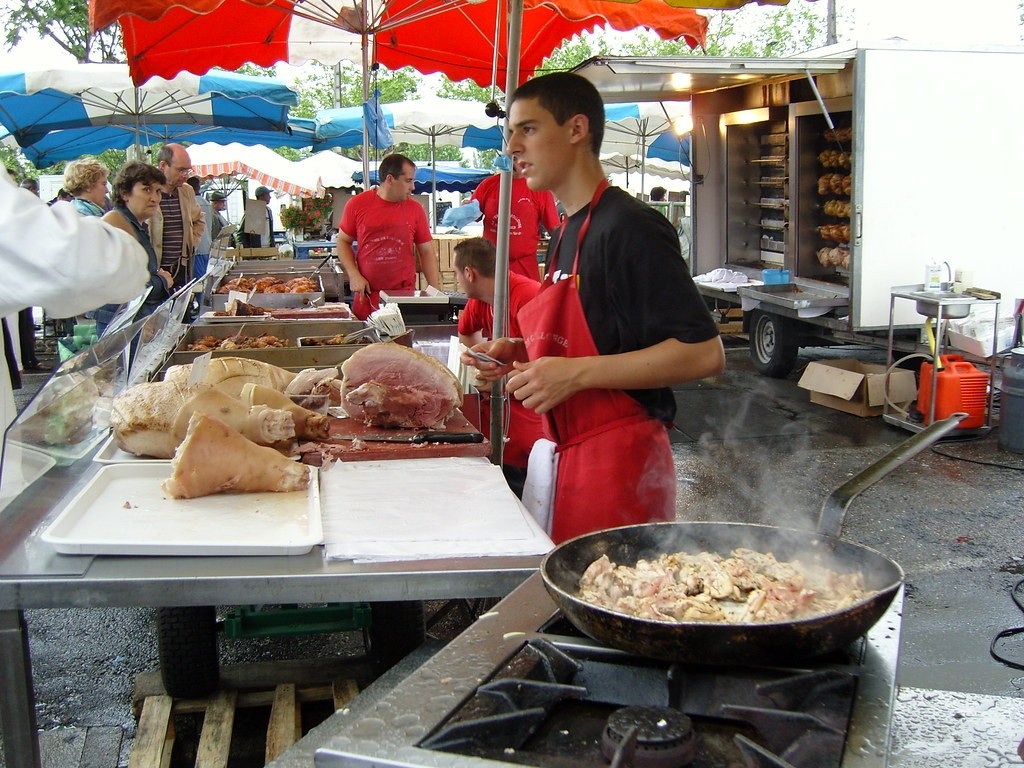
[885,281,1002,440]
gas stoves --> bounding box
[310,566,904,768]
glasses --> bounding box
[174,168,196,178]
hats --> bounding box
[255,185,275,196]
[208,190,227,201]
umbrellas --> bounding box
[0,0,709,234]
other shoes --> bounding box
[21,362,53,373]
[84,313,94,319]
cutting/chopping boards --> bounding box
[301,407,492,460]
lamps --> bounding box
[671,112,710,185]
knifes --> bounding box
[330,430,483,443]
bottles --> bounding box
[997,347,1024,453]
[925,255,941,291]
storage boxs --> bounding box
[224,263,345,297]
[790,352,916,421]
[211,266,326,311]
[945,324,1015,359]
[150,347,366,383]
[171,316,387,352]
[225,245,279,259]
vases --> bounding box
[292,226,304,242]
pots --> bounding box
[539,410,969,661]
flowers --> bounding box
[279,194,336,229]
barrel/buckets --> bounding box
[916,353,988,430]
[762,268,790,285]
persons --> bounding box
[0,143,237,512]
[454,235,547,469]
[238,185,276,260]
[460,154,561,284]
[649,186,667,217]
[336,155,443,321]
[460,73,726,546]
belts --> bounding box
[160,262,186,274]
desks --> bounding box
[290,243,359,260]
